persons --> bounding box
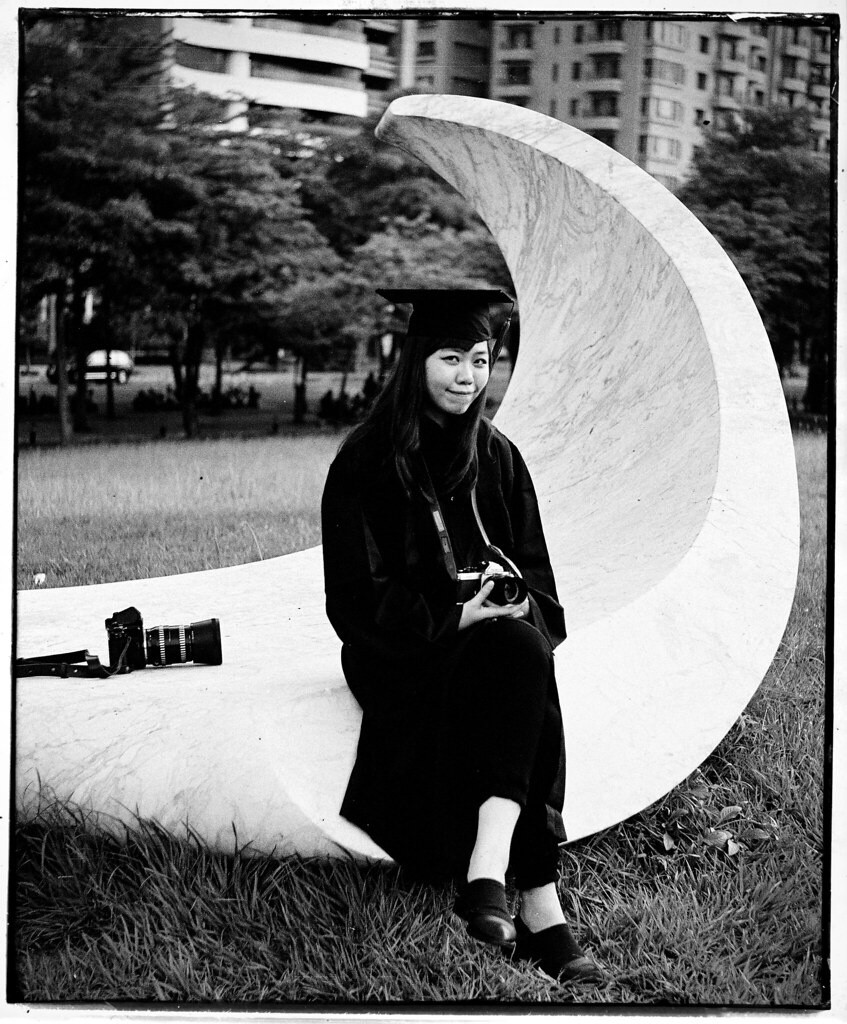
[320,289,607,982]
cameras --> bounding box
[105,606,222,672]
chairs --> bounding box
[11,93,803,862]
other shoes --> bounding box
[502,914,608,992]
[452,877,518,946]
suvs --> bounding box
[44,349,135,386]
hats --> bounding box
[374,287,515,353]
